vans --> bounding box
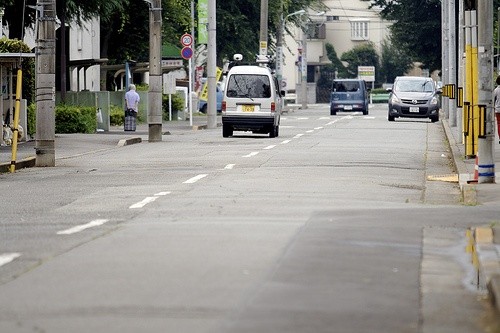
[329,78,371,116]
[222,64,287,139]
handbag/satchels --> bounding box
[125,109,137,118]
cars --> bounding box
[385,74,443,123]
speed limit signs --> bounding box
[181,34,193,46]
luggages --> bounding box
[124,108,136,131]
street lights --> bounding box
[278,10,307,92]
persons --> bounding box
[492,75,500,144]
[124,84,140,117]
